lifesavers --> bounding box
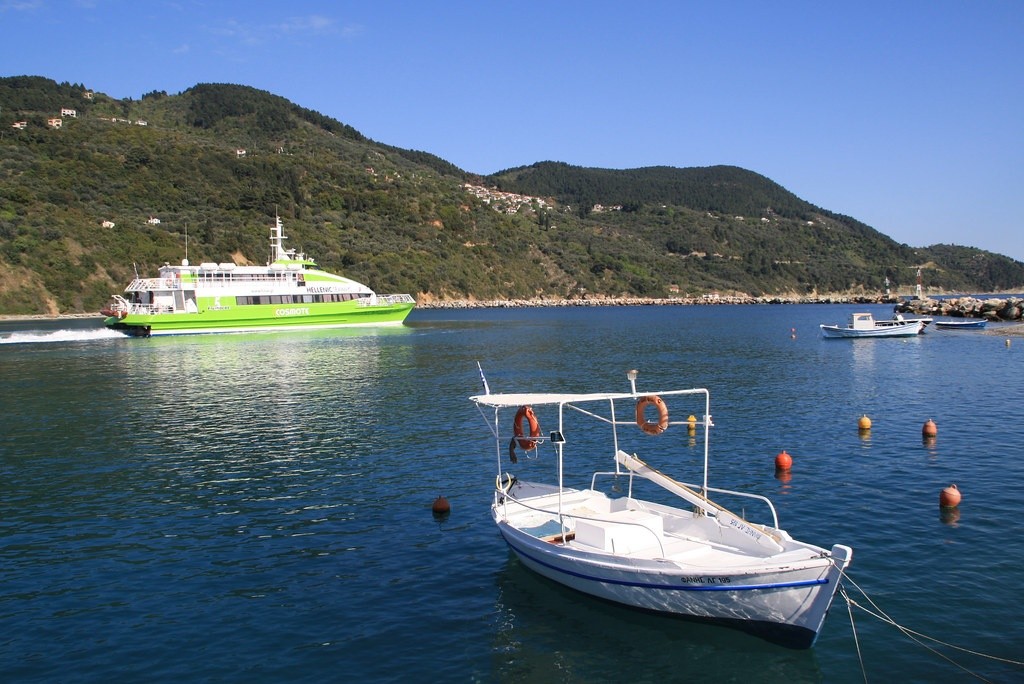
[636,395,669,436]
[512,407,541,452]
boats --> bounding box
[846,311,934,332]
[935,317,989,329]
[103,206,419,340]
[819,311,921,339]
[468,368,855,632]
